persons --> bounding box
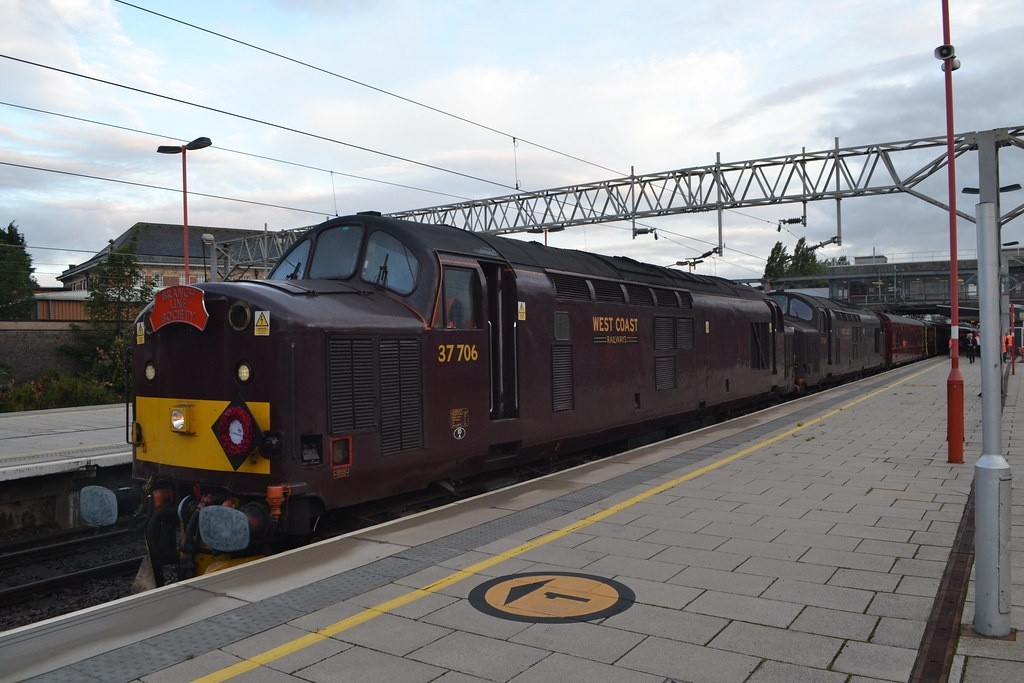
[1006,332,1013,359]
[965,333,980,364]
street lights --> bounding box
[200,234,217,282]
[157,136,212,284]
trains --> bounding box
[80,209,975,587]
